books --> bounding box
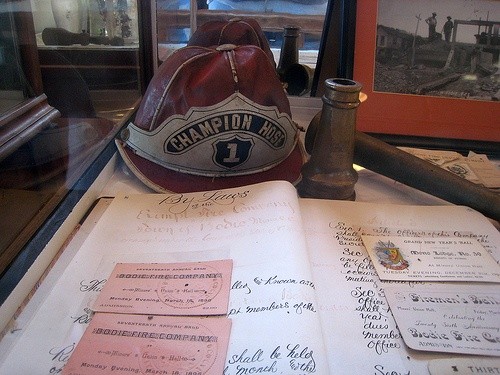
[0,177,500,375]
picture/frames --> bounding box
[349,0,500,146]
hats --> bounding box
[114,43,307,194]
[188,17,277,68]
